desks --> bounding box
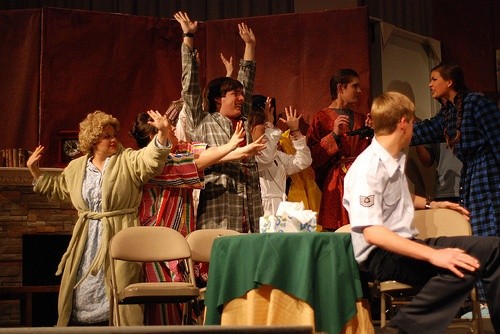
[202,232,375,333]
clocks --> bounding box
[51,130,86,168]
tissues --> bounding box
[259,199,318,233]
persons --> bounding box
[244,96,314,219]
[127,110,269,324]
[415,141,464,205]
[172,10,266,236]
[24,108,175,328]
[307,67,375,233]
[365,60,500,300]
[163,48,234,123]
[246,94,321,211]
[343,90,500,334]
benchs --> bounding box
[0,286,62,328]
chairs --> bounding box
[380,209,484,333]
[108,225,202,327]
[181,228,243,325]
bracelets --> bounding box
[182,32,194,38]
[290,128,301,133]
